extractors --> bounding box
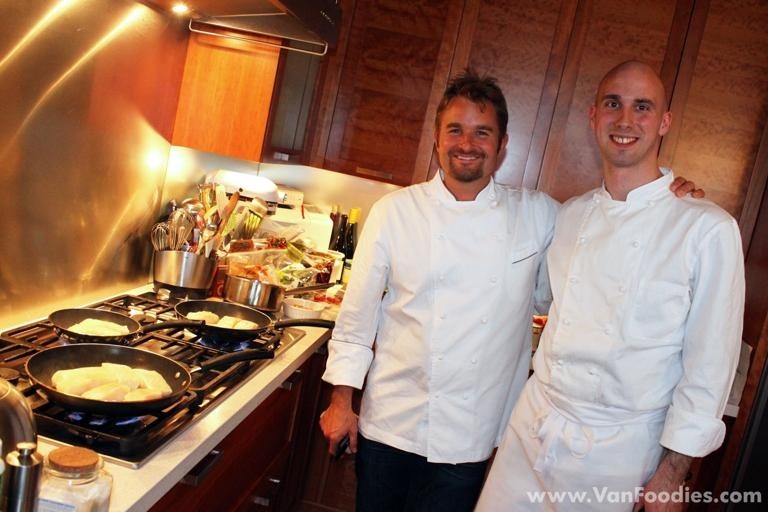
[135,0,343,57]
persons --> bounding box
[318,70,705,512]
[471,61,746,512]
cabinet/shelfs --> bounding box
[313,362,768,512]
[170,0,768,262]
[142,364,306,511]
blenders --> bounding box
[205,169,295,217]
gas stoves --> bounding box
[0,294,306,470]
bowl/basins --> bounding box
[155,250,215,291]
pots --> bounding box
[25,273,335,413]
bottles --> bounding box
[328,204,360,284]
[3,440,111,511]
